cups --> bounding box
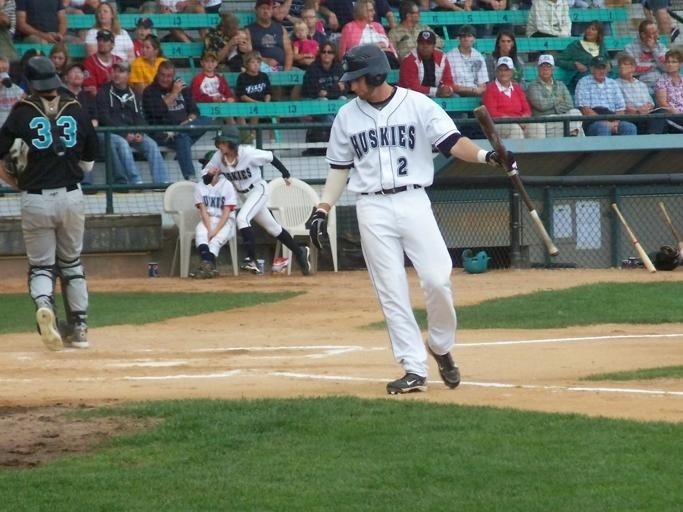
[257,259,264,276]
[147,264,158,275]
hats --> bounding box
[591,55,607,66]
[495,56,514,70]
[241,50,263,64]
[96,29,114,42]
[537,54,555,66]
[112,61,130,72]
[135,17,153,27]
[256,0,271,7]
[201,47,217,60]
[25,56,62,92]
[417,30,436,42]
[64,62,85,73]
[455,24,476,37]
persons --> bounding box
[2,0,682,201]
[305,42,519,397]
[0,57,103,351]
[189,147,237,278]
[201,123,312,277]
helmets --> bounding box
[199,149,217,169]
[654,245,680,271]
[338,44,391,82]
[461,248,491,274]
[210,125,241,145]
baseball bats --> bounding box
[610,203,657,273]
[473,105,561,257]
[657,201,683,258]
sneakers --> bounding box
[189,260,219,279]
[297,245,311,274]
[111,178,129,193]
[81,182,97,194]
[239,257,261,274]
[386,372,428,395]
[425,338,462,388]
[35,303,63,351]
[62,317,90,348]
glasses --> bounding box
[322,50,334,54]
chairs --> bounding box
[162,179,239,280]
[265,177,337,276]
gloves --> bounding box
[309,208,330,250]
[485,148,519,177]
